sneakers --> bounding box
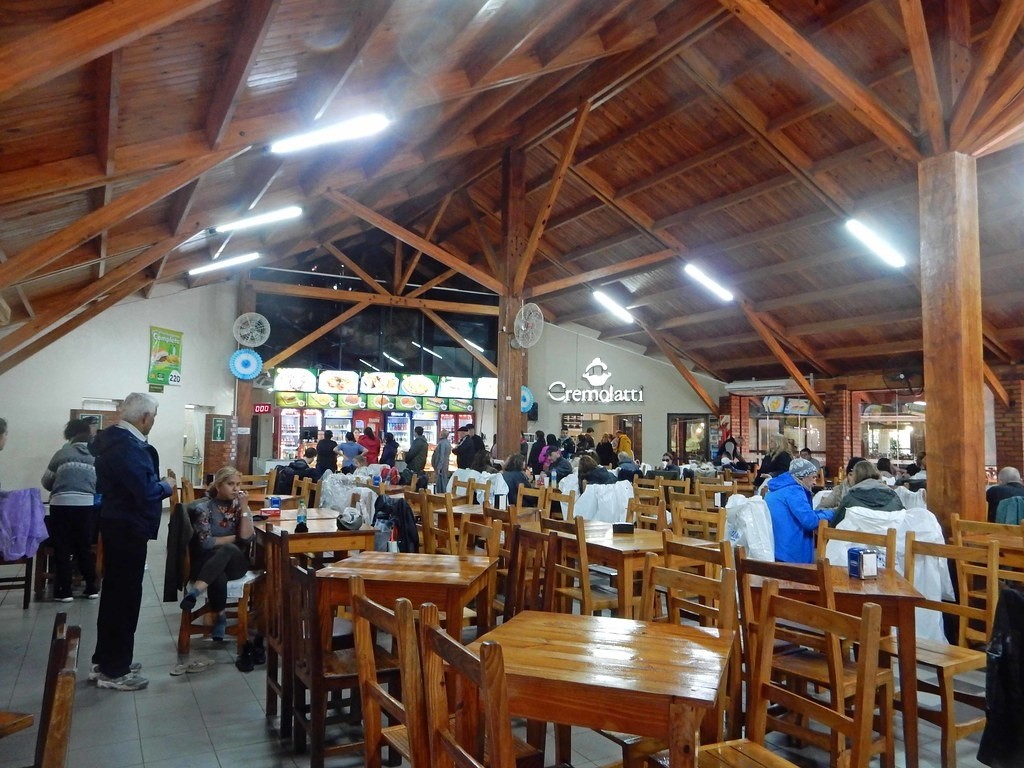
[89,661,149,691]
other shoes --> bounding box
[83,588,99,599]
[52,588,73,603]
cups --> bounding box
[329,402,336,407]
[544,477,549,488]
[467,405,473,411]
[535,475,541,488]
[441,404,447,411]
[415,404,421,410]
[359,402,365,408]
[298,400,305,407]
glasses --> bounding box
[662,459,669,462]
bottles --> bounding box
[281,424,296,433]
[297,500,307,524]
[551,469,557,488]
[281,437,296,446]
[389,422,408,430]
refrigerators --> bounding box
[386,410,412,455]
[324,409,353,443]
[457,411,475,443]
[301,409,325,431]
[273,407,302,459]
[440,412,457,442]
[412,410,438,443]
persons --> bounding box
[333,432,369,475]
[752,434,792,495]
[578,455,618,494]
[316,430,338,479]
[721,451,749,481]
[353,427,380,466]
[432,429,451,493]
[658,452,680,476]
[764,459,837,562]
[0,418,8,450]
[41,419,100,602]
[815,457,903,550]
[520,430,529,464]
[986,466,1024,523]
[405,426,428,472]
[470,449,499,505]
[294,447,317,468]
[618,451,640,471]
[491,434,498,468]
[902,452,927,492]
[88,393,177,690]
[452,424,485,469]
[379,432,399,468]
[529,428,634,481]
[796,448,820,478]
[544,446,573,483]
[496,454,538,507]
[179,466,257,642]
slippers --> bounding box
[170,660,215,676]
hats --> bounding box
[341,507,363,530]
[789,459,818,477]
[83,417,98,424]
[543,446,558,456]
[457,427,468,432]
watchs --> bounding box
[240,513,250,518]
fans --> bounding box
[510,303,544,349]
[233,312,270,347]
[882,355,924,398]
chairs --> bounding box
[826,504,956,644]
[951,512,1024,649]
[894,485,927,510]
[0,486,50,610]
[879,529,999,768]
[735,544,895,768]
[815,519,897,711]
[22,612,83,768]
[71,530,103,591]
[163,465,802,768]
[995,495,1024,587]
[699,579,883,768]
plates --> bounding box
[400,396,416,408]
[274,368,316,392]
[319,370,358,394]
[373,395,389,407]
[280,394,299,405]
[387,403,394,409]
[401,375,434,396]
[310,393,334,405]
[342,395,361,405]
[360,373,399,395]
[864,405,882,416]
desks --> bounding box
[253,517,382,639]
[443,609,737,768]
[559,529,717,627]
[390,493,468,547]
[749,562,926,768]
[248,493,305,510]
[531,482,562,519]
[665,499,718,534]
[251,506,340,568]
[949,533,1024,645]
[434,502,539,554]
[33,504,50,592]
[315,550,499,714]
[520,518,608,550]
[193,484,268,511]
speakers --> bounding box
[527,402,538,421]
